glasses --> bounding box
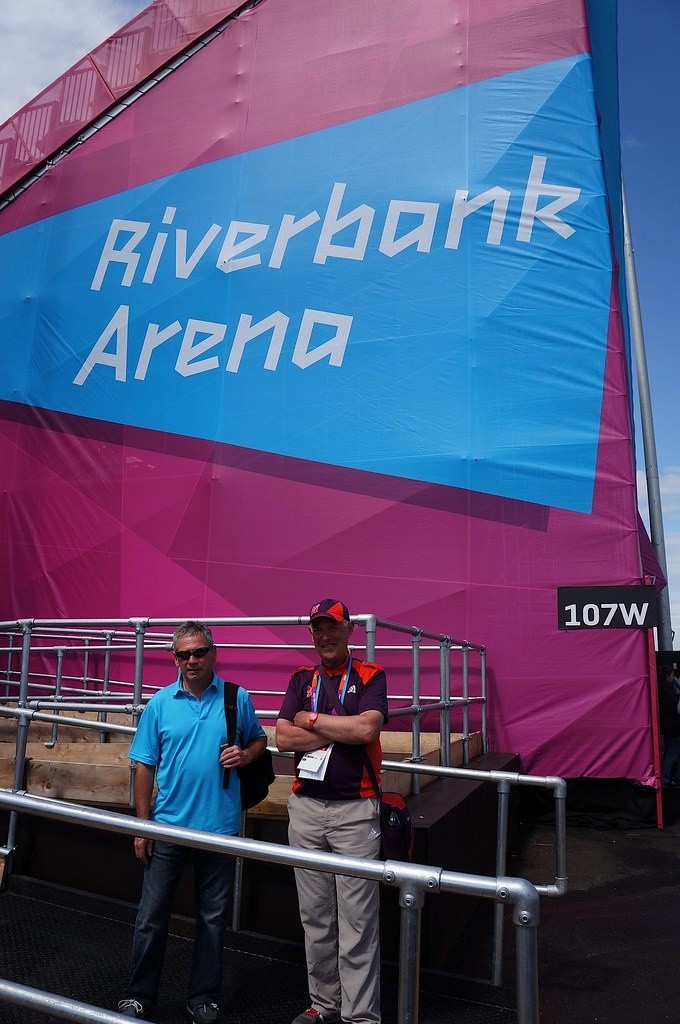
[175,647,212,661]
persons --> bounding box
[117,620,268,1024]
[274,598,390,1024]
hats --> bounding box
[308,599,350,634]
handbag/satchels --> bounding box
[379,791,415,863]
[222,681,276,811]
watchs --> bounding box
[308,712,318,730]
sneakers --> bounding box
[175,994,217,1024]
[117,998,148,1019]
[291,1008,341,1024]
